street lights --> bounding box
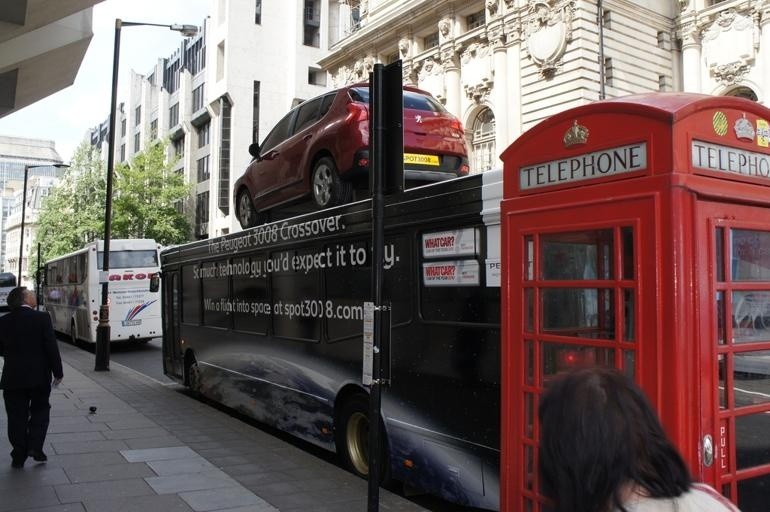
[18,160,73,288]
[94,19,200,372]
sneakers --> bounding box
[11,455,26,468]
[27,448,47,461]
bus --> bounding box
[35,234,166,352]
[150,166,734,512]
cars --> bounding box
[228,79,472,232]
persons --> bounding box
[537,360,742,512]
[0,285,65,470]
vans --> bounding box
[0,271,18,307]
